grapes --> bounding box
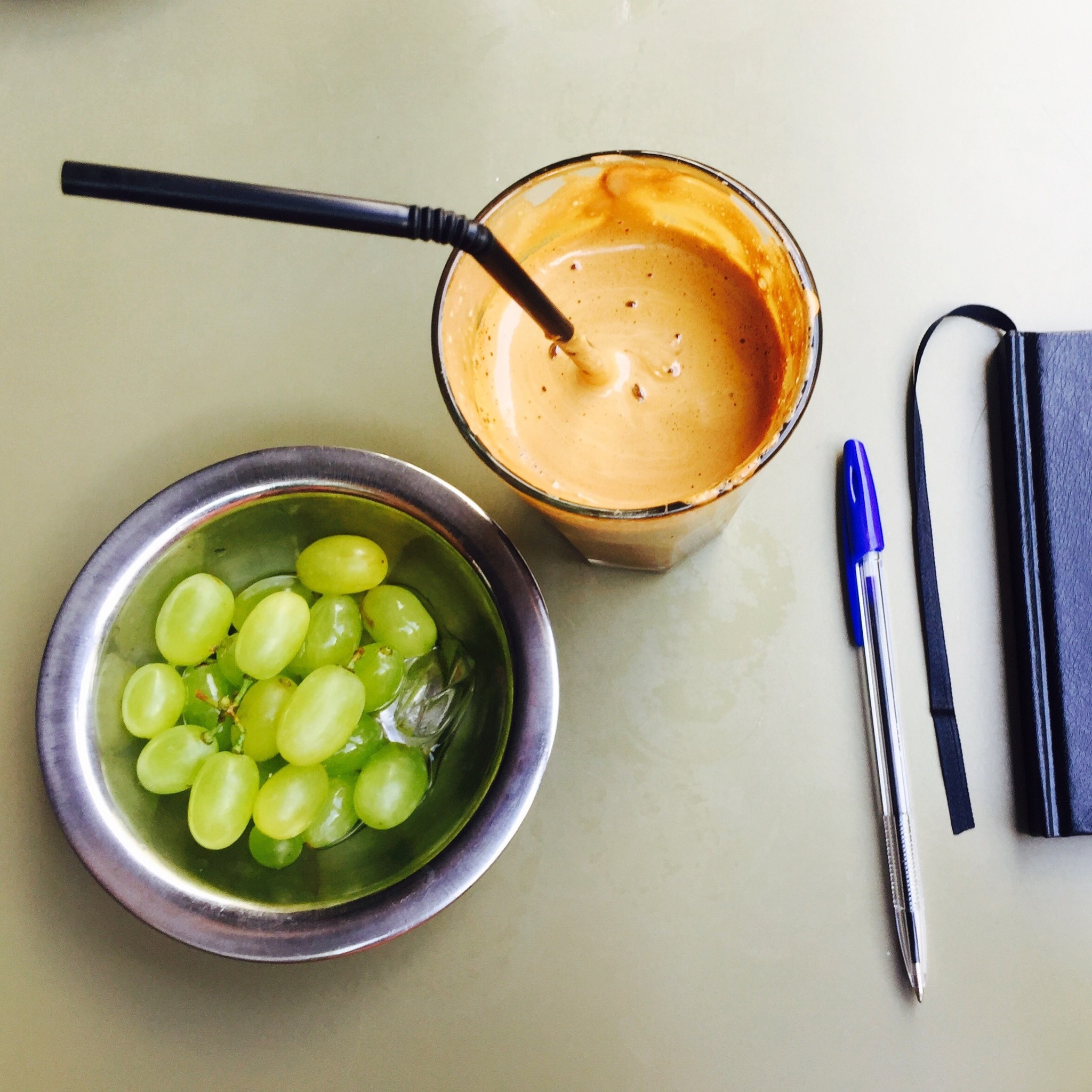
[121,535,437,868]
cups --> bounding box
[432,150,822,573]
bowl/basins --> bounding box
[35,448,560,963]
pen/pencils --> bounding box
[835,439,930,1002]
